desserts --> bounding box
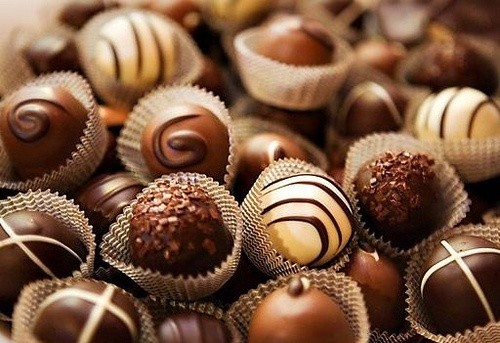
[1,1,500,343]
